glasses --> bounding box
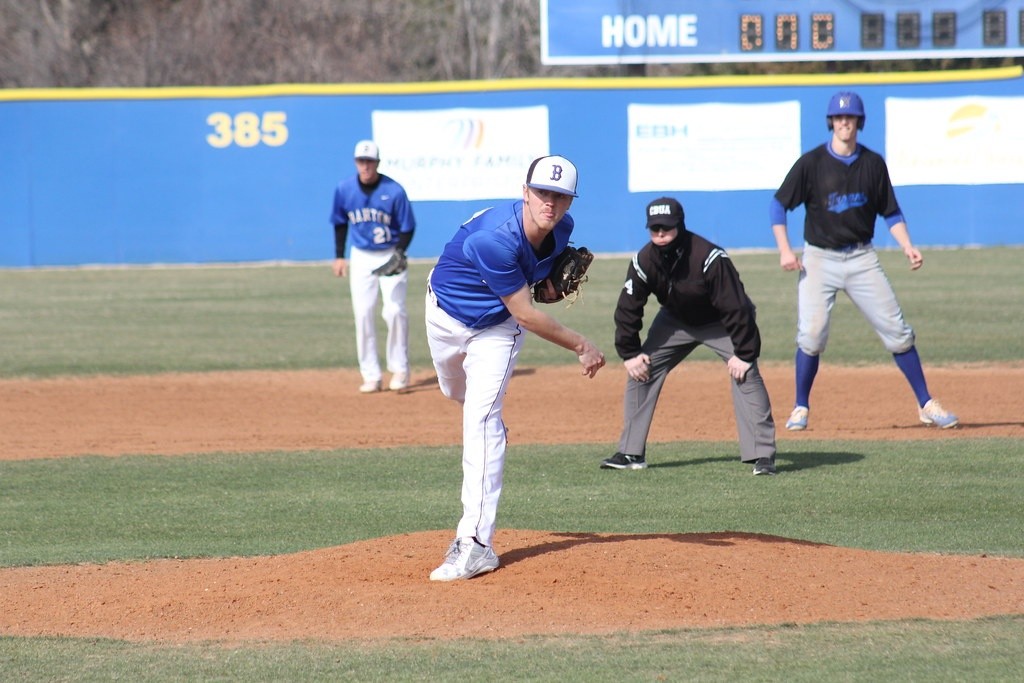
[650,224,676,232]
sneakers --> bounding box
[917,399,959,428]
[429,537,500,581]
[752,458,777,475]
[600,451,647,469]
[785,405,810,431]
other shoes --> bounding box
[389,371,409,390]
[359,380,383,393]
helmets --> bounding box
[826,91,865,131]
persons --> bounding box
[599,197,776,475]
[769,92,958,431]
[328,139,415,392]
[425,153,605,583]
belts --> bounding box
[835,240,872,252]
[427,286,439,307]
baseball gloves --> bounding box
[371,249,408,277]
[529,240,595,310]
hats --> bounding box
[646,197,684,229]
[526,154,579,197]
[354,139,380,160]
